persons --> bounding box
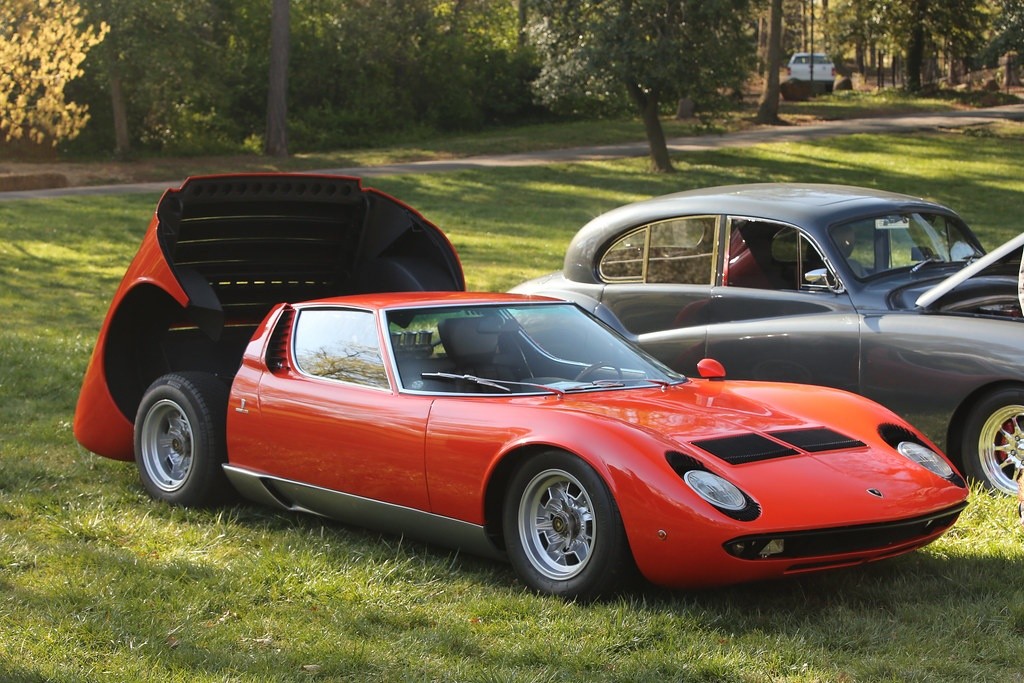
[832,226,869,278]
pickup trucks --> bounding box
[786,54,837,84]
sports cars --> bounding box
[72,170,972,601]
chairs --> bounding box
[731,239,793,290]
[437,317,523,394]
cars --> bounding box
[500,180,1024,496]
